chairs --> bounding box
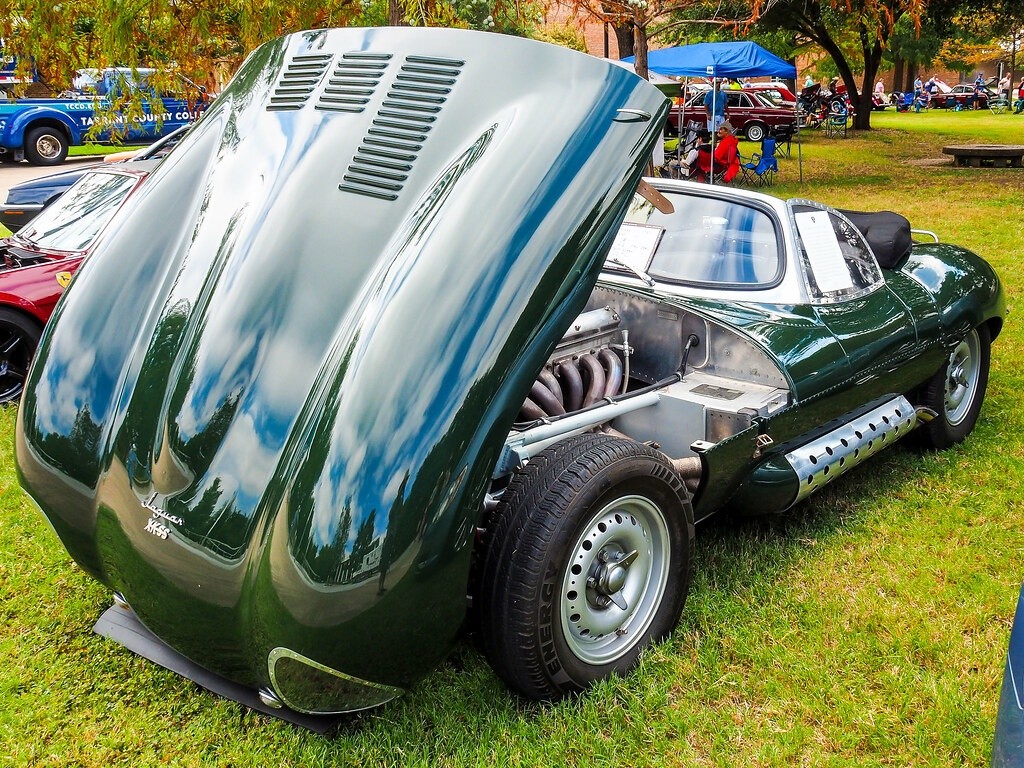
[811,91,1024,139]
[660,120,799,188]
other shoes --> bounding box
[680,161,690,168]
[977,107,981,110]
[659,167,670,178]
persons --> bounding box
[829,76,839,93]
[889,88,905,111]
[875,78,891,106]
[805,100,827,127]
[914,75,935,113]
[998,72,1011,110]
[668,118,740,183]
[802,75,814,88]
[675,75,691,105]
[973,73,984,110]
[1013,77,1024,114]
[703,77,752,147]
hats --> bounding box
[832,77,840,80]
[879,78,884,82]
[696,128,710,137]
[712,77,723,84]
[720,122,732,131]
[805,75,810,80]
[978,72,983,75]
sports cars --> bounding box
[12,26,1011,735]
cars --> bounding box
[744,86,798,107]
[0,71,218,165]
[0,159,166,406]
[662,89,796,141]
[0,120,198,233]
[929,82,998,109]
